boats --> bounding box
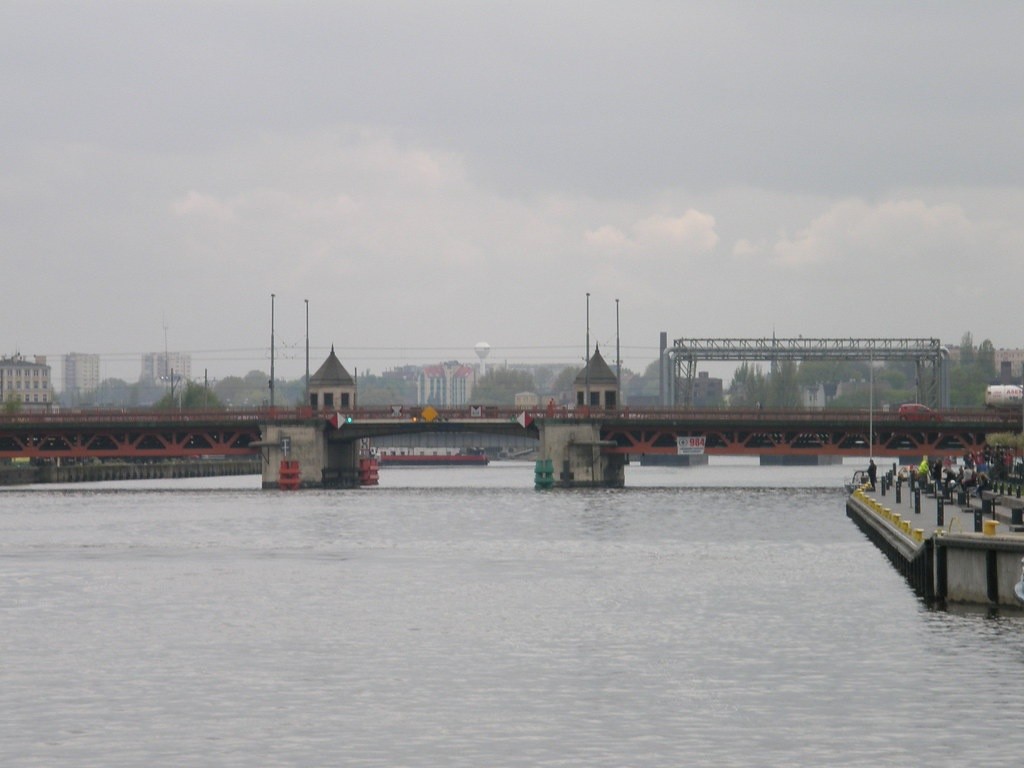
[379,445,490,466]
[846,470,869,493]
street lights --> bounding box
[270,294,275,406]
[615,298,621,410]
[305,299,310,406]
[586,293,590,407]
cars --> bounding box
[983,444,1014,473]
[899,404,943,422]
[937,464,963,488]
[30,436,80,464]
[898,466,919,481]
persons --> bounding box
[867,459,877,492]
[973,472,993,499]
[919,459,929,490]
[941,468,956,488]
[943,469,964,499]
[933,460,942,490]
